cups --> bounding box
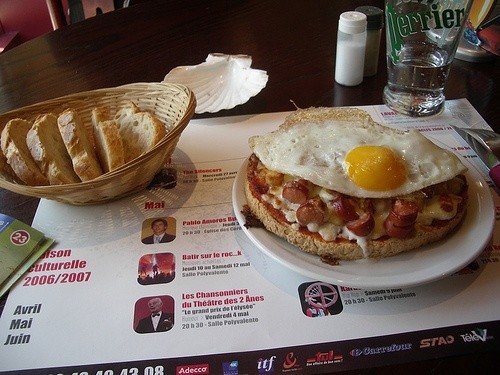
[383,0,474,117]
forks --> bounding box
[460,128,500,162]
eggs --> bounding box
[248,107,468,199]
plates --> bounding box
[232,133,495,290]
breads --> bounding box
[1,101,167,187]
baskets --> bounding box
[0,83,197,205]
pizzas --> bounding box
[246,153,469,260]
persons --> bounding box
[135,299,173,332]
[142,218,176,244]
[304,298,331,318]
[138,257,174,284]
[156,168,176,190]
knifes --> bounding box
[449,123,500,190]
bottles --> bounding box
[334,6,383,85]
